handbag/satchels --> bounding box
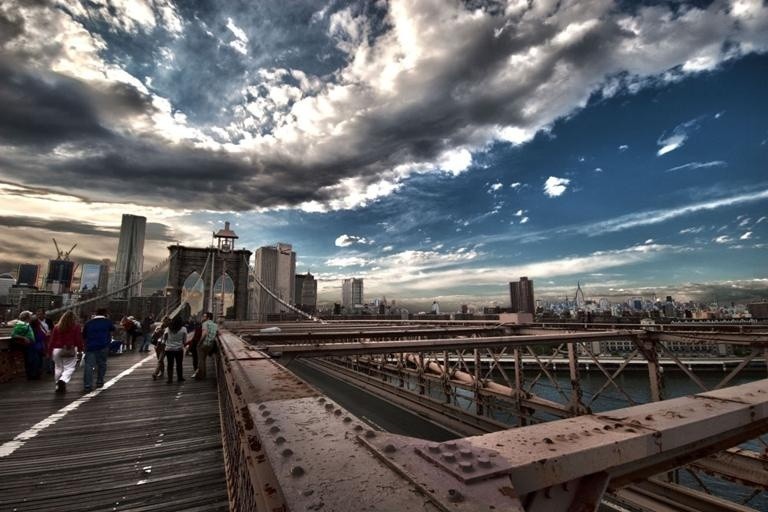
[59,345,75,357]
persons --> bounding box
[36,307,53,343]
[46,311,83,394]
[82,308,115,393]
[11,311,44,381]
[112,313,216,383]
